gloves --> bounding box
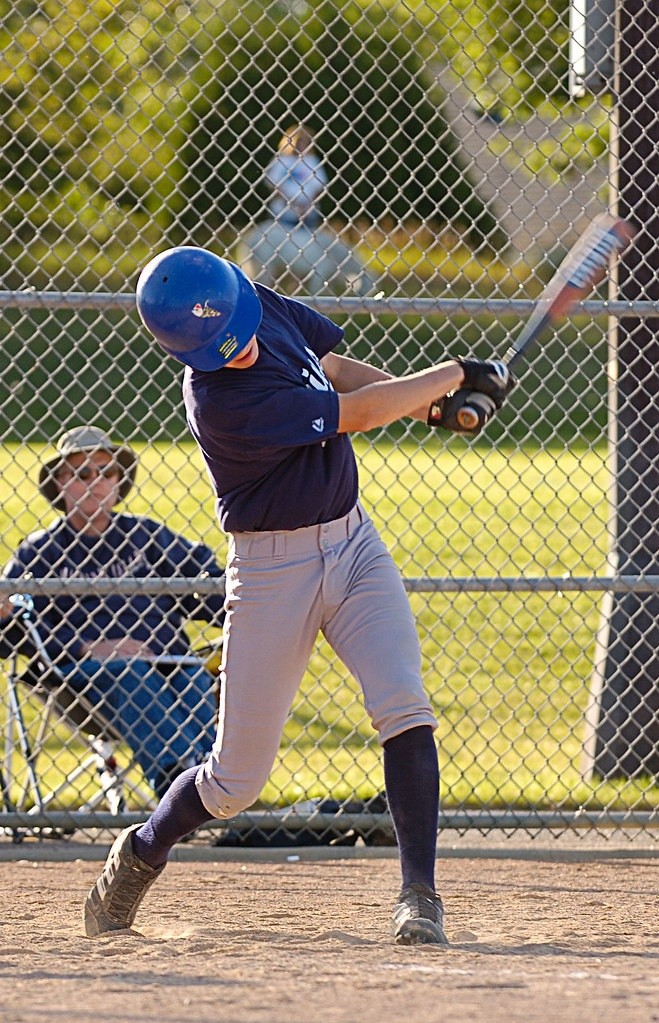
[427,355,516,434]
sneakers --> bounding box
[390,882,449,947]
[84,823,167,937]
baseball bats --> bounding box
[457,212,635,428]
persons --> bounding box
[84,246,515,947]
[235,124,376,293]
[0,425,227,850]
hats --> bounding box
[39,425,137,512]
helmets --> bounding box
[136,246,264,372]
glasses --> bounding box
[59,466,120,481]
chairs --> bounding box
[0,593,223,838]
